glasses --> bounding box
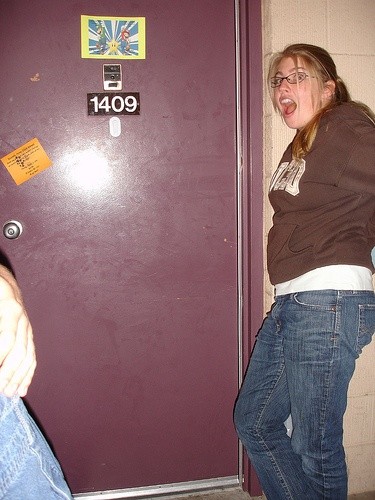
[270,72,317,88]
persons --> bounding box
[231,43,375,499]
[0,265,75,500]
